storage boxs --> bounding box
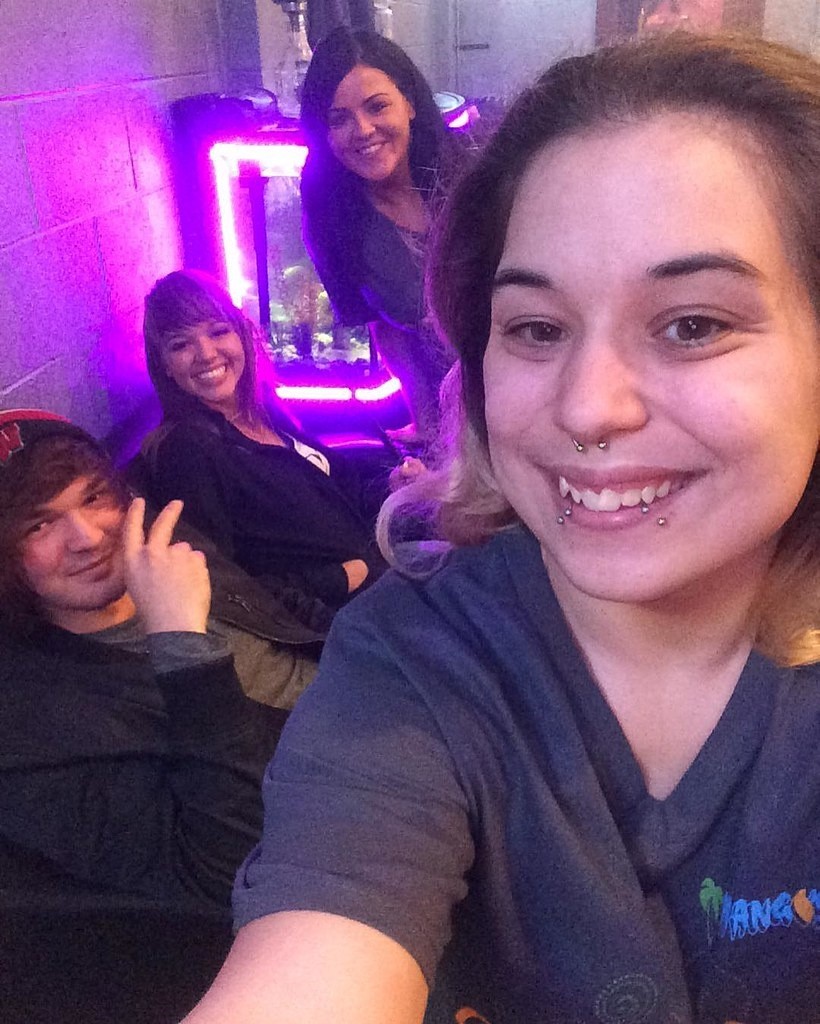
[200,104,480,434]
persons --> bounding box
[182,31,820,1024]
[133,268,428,610]
[0,408,327,917]
[299,26,474,436]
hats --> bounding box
[0,405,106,472]
